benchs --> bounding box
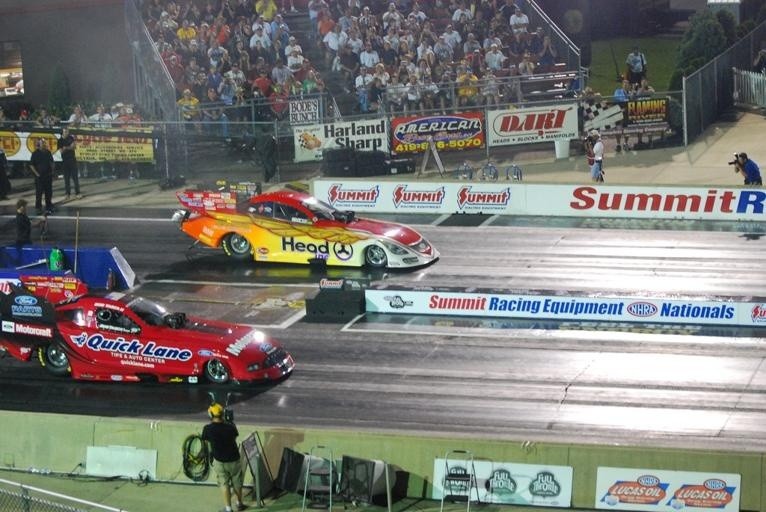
[126,0,582,137]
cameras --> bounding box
[584,135,593,141]
[729,153,738,164]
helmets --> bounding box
[207,402,223,418]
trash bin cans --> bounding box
[554,138,571,159]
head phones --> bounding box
[36,138,42,147]
[208,403,225,419]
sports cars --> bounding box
[0,274,296,386]
[172,181,440,269]
[322,149,416,176]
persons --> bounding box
[14,199,42,251]
[586,130,605,183]
[1,105,145,211]
[202,403,248,512]
[624,46,647,83]
[258,126,277,181]
[735,153,762,185]
[636,79,655,96]
[613,80,634,102]
[308,1,556,119]
[138,1,325,137]
